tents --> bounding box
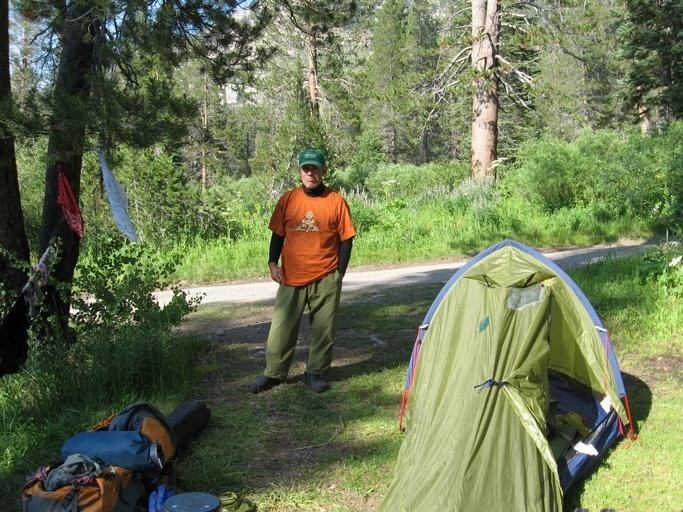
[376,237,636,512]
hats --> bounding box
[299,149,326,169]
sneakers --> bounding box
[304,373,331,393]
[250,375,283,393]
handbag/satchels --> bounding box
[21,399,210,512]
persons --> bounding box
[244,148,357,392]
